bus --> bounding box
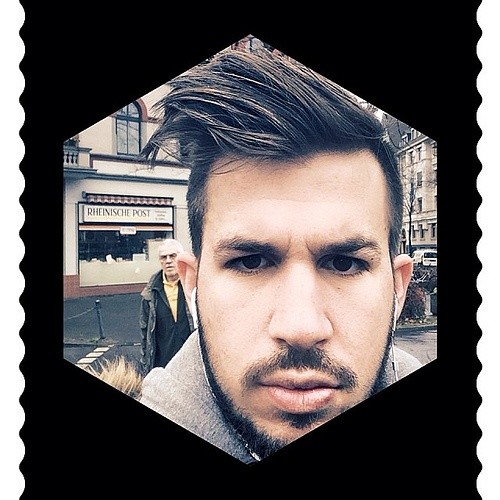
[409,249,438,269]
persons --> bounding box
[127,40,427,465]
[138,238,201,378]
